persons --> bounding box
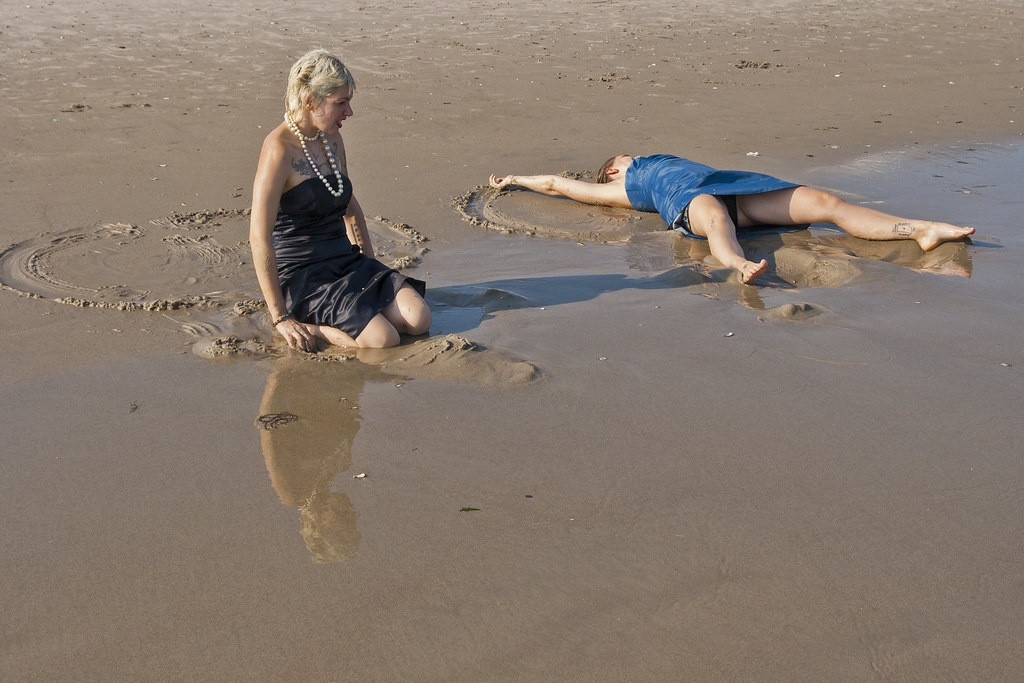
[259,351,415,567]
[489,153,975,284]
[249,47,433,353]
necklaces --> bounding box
[283,112,343,197]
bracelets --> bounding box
[510,175,514,185]
[272,314,289,327]
